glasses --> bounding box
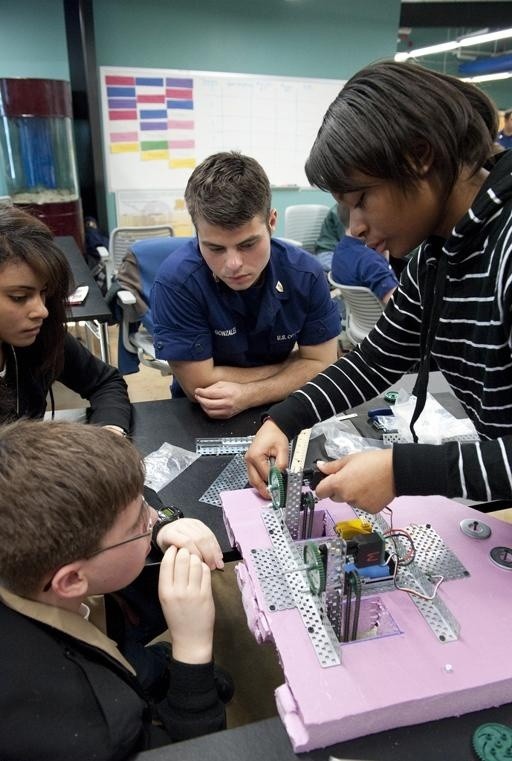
[44,495,153,590]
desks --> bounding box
[49,234,114,363]
[43,395,511,760]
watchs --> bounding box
[151,505,184,551]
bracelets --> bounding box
[104,427,127,438]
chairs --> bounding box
[327,271,385,359]
[271,204,346,327]
[89,224,173,298]
[110,271,172,379]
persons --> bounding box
[152,150,342,419]
[496,109,512,151]
[83,216,109,261]
[313,201,346,272]
[0,201,132,440]
[330,201,399,306]
[0,418,234,761]
[244,60,512,513]
